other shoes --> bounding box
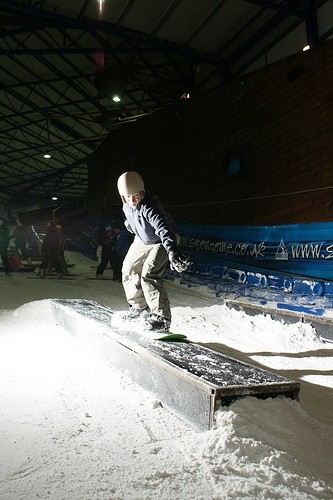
[118,307,150,322]
[139,314,170,332]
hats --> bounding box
[117,172,145,204]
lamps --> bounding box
[43,148,51,158]
[177,91,190,98]
[112,88,121,103]
[52,192,58,200]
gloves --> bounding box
[167,248,194,272]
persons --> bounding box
[96,225,129,282]
[39,224,68,279]
[9,223,30,252]
[117,172,190,333]
[0,218,12,277]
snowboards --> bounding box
[64,262,76,267]
[27,272,80,281]
[112,311,188,340]
[89,265,113,270]
[86,275,118,281]
